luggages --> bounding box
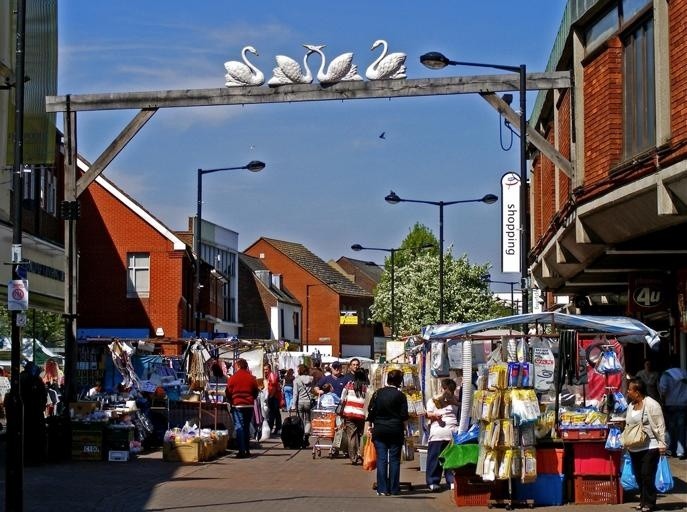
[279,407,305,449]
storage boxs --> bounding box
[574,443,616,477]
[511,474,564,505]
[163,435,227,463]
[535,449,564,474]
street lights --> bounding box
[385,191,498,323]
[306,281,342,352]
[420,52,531,336]
[351,244,434,339]
[482,279,518,315]
[192,160,265,339]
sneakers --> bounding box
[635,501,653,511]
[377,491,399,497]
[426,482,443,492]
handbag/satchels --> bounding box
[336,399,345,416]
[360,438,379,469]
[621,424,647,448]
[332,424,348,452]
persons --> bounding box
[19,360,48,465]
[224,359,259,459]
[657,352,687,460]
[634,356,662,407]
[363,369,409,496]
[85,379,103,396]
[250,357,370,466]
[624,376,668,512]
[40,357,64,386]
[425,389,459,491]
[425,377,461,433]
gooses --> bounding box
[267,39,327,87]
[222,44,266,88]
[301,44,360,82]
[363,37,407,82]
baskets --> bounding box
[452,476,491,507]
[572,477,623,504]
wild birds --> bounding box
[379,131,386,139]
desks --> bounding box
[153,399,236,440]
[440,439,623,505]
[47,423,134,463]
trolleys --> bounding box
[310,393,339,459]
